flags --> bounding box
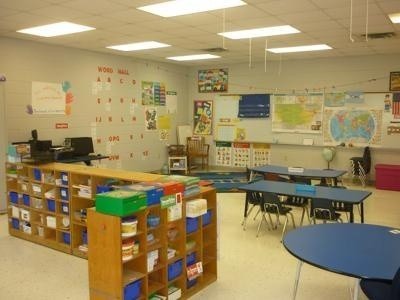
[391,92,400,115]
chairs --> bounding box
[186,136,211,174]
[215,137,398,170]
[350,146,374,187]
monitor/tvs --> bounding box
[238,94,270,118]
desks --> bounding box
[239,164,400,300]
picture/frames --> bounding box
[389,71,400,91]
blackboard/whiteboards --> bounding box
[213,92,392,148]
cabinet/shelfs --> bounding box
[4,161,217,300]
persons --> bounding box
[194,112,211,135]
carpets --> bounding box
[183,170,312,193]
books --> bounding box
[149,284,183,300]
[147,246,176,272]
[288,166,304,174]
